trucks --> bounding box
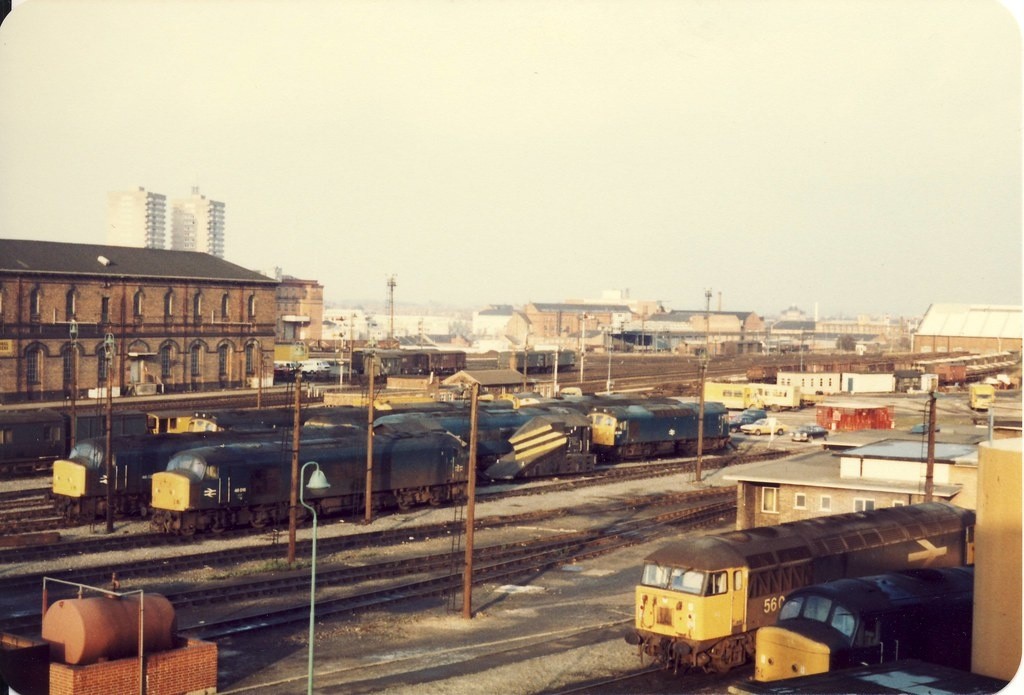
[749,384,800,413]
[800,393,824,409]
[704,383,764,410]
[969,384,995,412]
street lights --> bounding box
[300,461,331,694]
[69,320,79,449]
[339,332,344,387]
[580,313,589,383]
[350,313,358,381]
[103,333,115,534]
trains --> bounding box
[754,564,974,682]
[351,348,466,378]
[1,393,732,538]
[498,350,577,374]
[624,501,974,680]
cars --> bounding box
[741,409,767,421]
[790,424,828,443]
[912,424,940,435]
[729,416,754,433]
[740,419,788,435]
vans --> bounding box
[302,361,331,374]
